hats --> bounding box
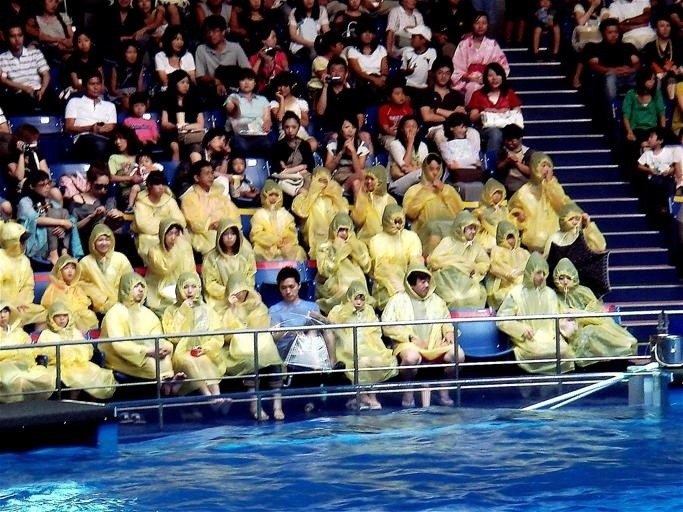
[407,25,432,42]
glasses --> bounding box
[38,180,52,186]
[95,184,109,190]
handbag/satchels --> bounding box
[271,172,304,197]
[178,132,205,145]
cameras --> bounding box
[24,144,37,152]
[265,46,272,53]
[326,76,342,84]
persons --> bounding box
[0,0,682,427]
